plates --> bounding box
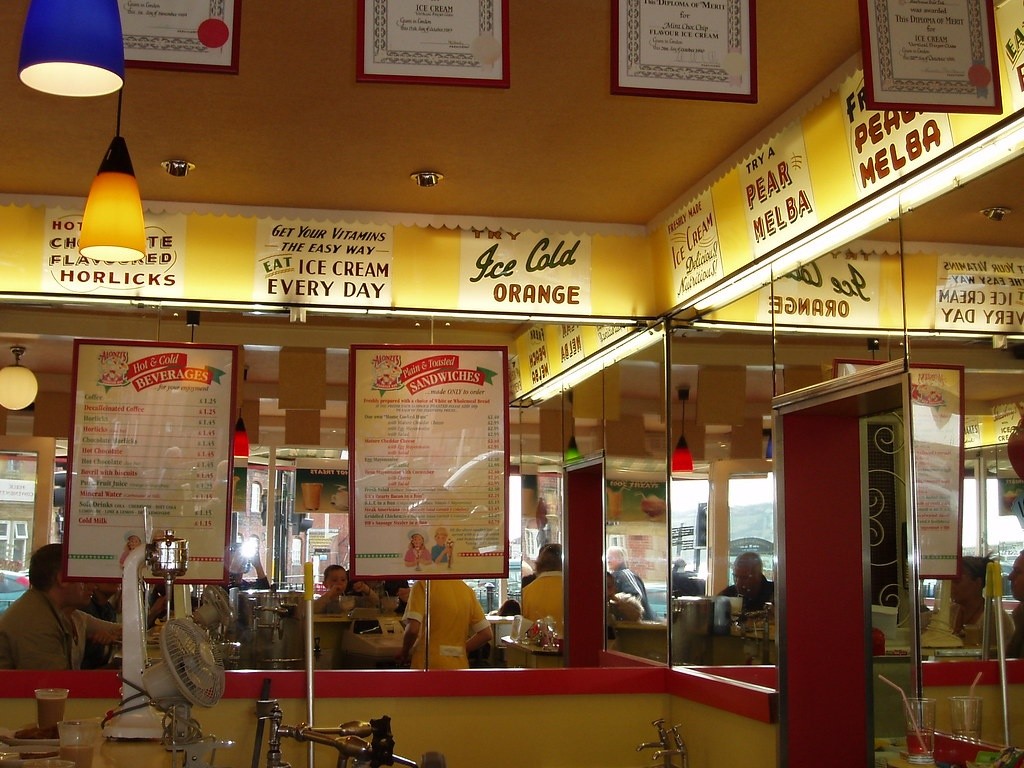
[0,726,75,768]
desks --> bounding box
[104,640,163,670]
[472,610,521,664]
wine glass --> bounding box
[381,596,399,616]
[338,595,355,617]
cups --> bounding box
[902,697,936,764]
[948,696,982,739]
[301,483,323,510]
[56,720,95,768]
[33,688,69,730]
[730,597,743,635]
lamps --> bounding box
[765,433,773,459]
[0,344,40,413]
[233,411,251,458]
[77,84,149,262]
[564,388,583,461]
[14,0,127,99]
[668,380,699,476]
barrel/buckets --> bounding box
[671,595,715,666]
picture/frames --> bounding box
[607,0,760,106]
[860,0,1006,117]
[355,0,513,90]
[117,0,244,77]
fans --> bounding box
[137,611,238,754]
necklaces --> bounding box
[960,599,982,628]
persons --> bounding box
[920,549,1024,659]
[488,542,654,659]
[310,564,412,670]
[716,551,775,623]
[79,532,271,670]
[670,558,697,599]
[395,578,491,668]
[0,543,169,669]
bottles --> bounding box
[510,614,560,651]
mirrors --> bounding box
[772,185,906,397]
[0,297,668,670]
[909,152,1024,757]
[667,259,775,687]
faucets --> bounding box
[636,717,672,768]
[651,723,689,768]
[313,637,321,663]
